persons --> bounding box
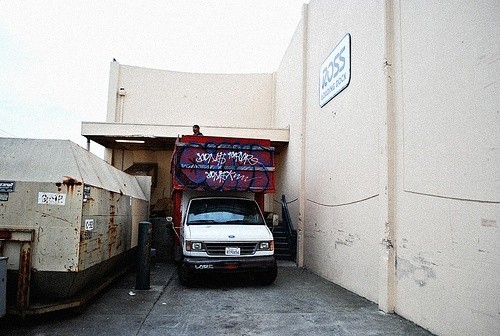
[192,125,203,136]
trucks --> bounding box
[165,134,280,285]
